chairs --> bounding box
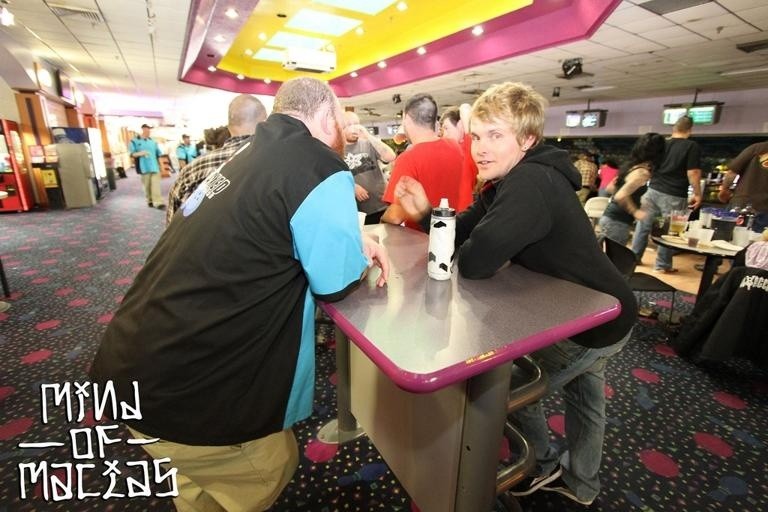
[600,235,676,326]
[584,195,609,235]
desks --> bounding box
[651,232,762,323]
[314,223,622,512]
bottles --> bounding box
[52,128,75,144]
[710,168,720,184]
[699,203,754,242]
[427,197,458,281]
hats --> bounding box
[140,124,153,129]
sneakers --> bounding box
[543,478,594,506]
[510,465,561,496]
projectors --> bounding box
[282,51,335,73]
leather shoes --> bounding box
[694,264,706,270]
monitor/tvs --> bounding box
[687,101,722,125]
[565,110,583,127]
[662,102,688,124]
[582,110,607,127]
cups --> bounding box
[392,124,408,145]
[639,296,658,316]
[668,208,714,248]
[145,152,150,158]
[358,212,366,230]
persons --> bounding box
[175,124,230,169]
[572,115,768,391]
[86,78,389,512]
[165,94,267,229]
[128,123,167,210]
[341,93,478,234]
[393,80,638,506]
